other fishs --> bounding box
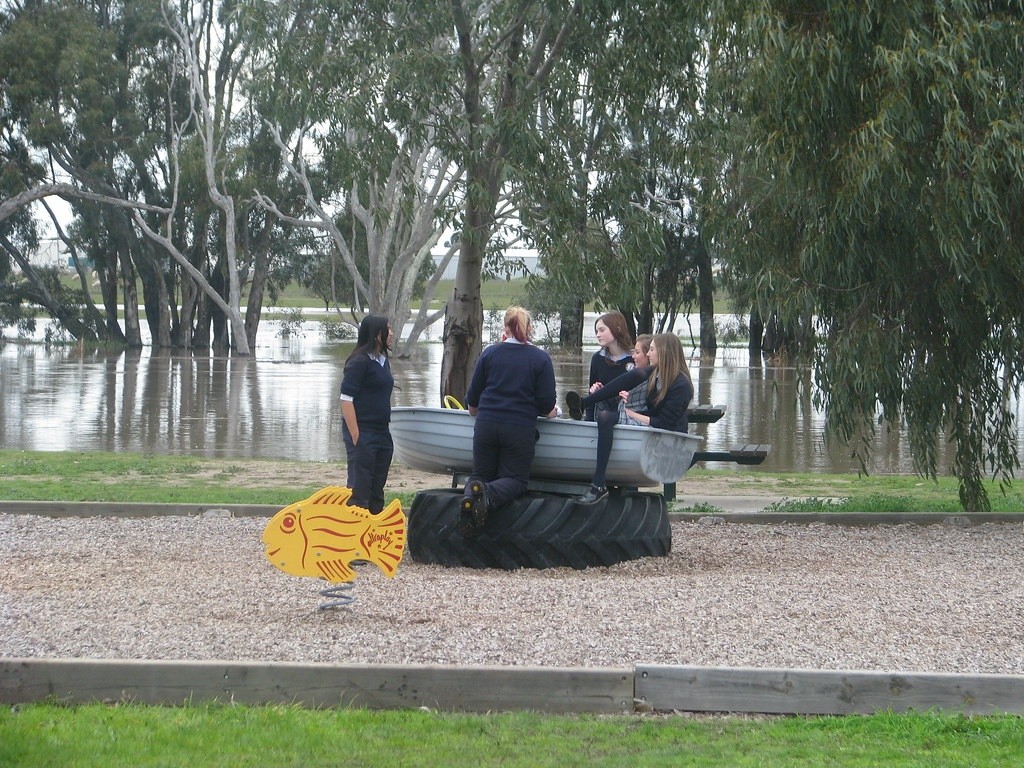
[262,485,406,583]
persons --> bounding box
[585,313,634,421]
[566,333,694,504]
[340,315,394,565]
[460,304,558,539]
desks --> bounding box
[663,404,727,500]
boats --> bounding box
[388,406,704,487]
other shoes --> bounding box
[350,559,367,566]
[566,390,582,421]
[458,497,474,539]
[576,482,609,505]
[469,480,488,528]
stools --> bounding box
[728,443,772,466]
